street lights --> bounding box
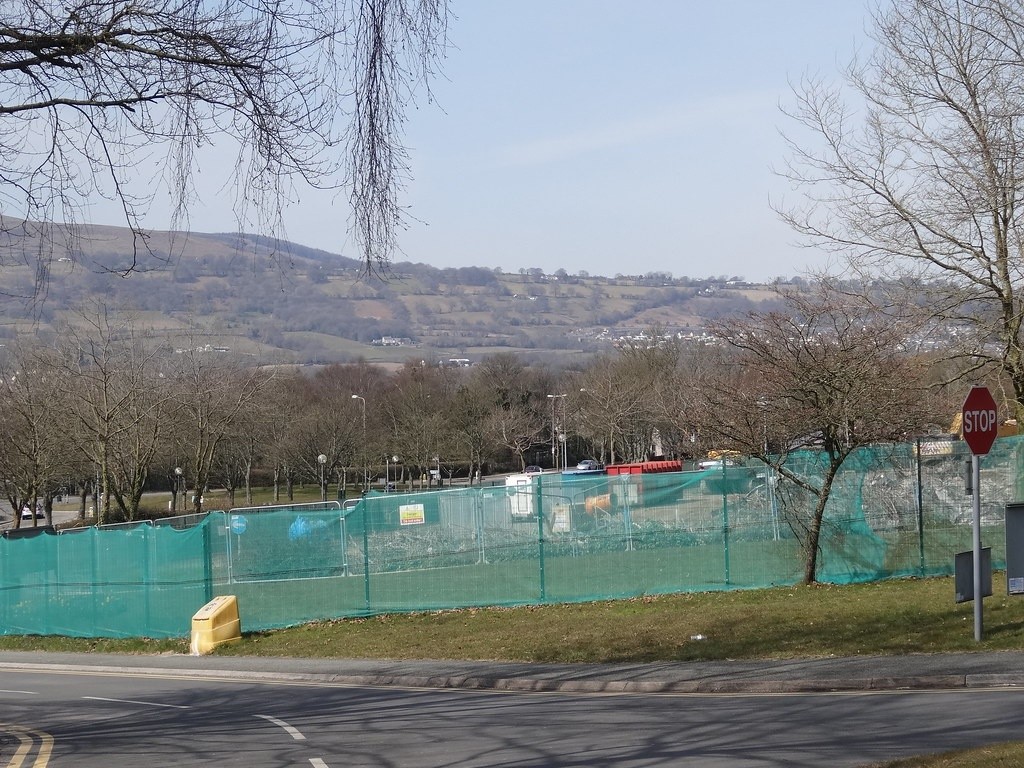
[548,394,568,472]
[392,455,399,493]
[351,394,366,492]
[175,467,183,517]
[319,454,328,502]
[559,433,567,470]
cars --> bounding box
[23,502,45,519]
[577,460,598,471]
[521,465,544,473]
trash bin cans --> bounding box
[189,595,242,656]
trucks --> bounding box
[505,469,608,523]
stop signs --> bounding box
[962,386,999,455]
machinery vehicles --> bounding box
[691,448,769,495]
[907,411,1023,467]
